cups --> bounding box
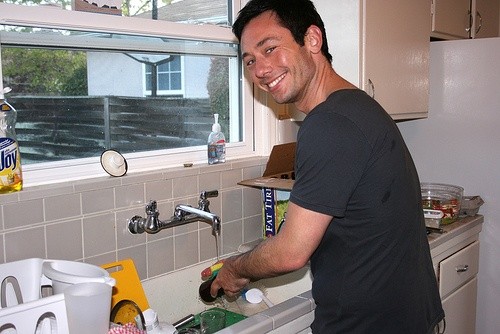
[198,309,226,334]
[173,327,200,334]
[63,281,113,334]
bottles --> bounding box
[0,87,24,195]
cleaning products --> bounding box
[207,113,226,165]
[0,86,23,195]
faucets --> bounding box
[173,203,221,237]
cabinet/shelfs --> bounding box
[430,223,480,334]
[431,0,500,40]
[292,0,431,122]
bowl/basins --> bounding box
[419,182,464,225]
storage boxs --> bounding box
[237,140,298,240]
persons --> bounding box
[210,0,445,334]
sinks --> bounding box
[140,251,314,334]
